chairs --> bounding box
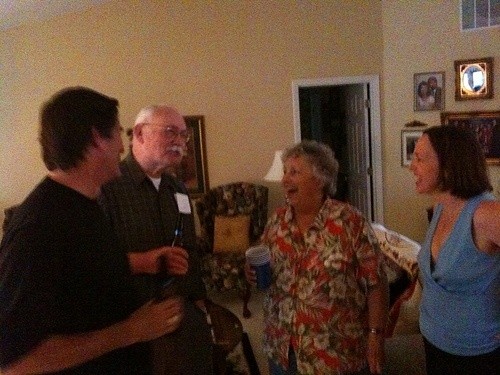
[196,182,268,320]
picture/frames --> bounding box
[454,57,494,101]
[400,130,425,167]
[414,70,445,111]
[441,110,500,165]
[179,115,210,199]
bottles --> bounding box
[171,212,186,249]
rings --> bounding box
[172,314,179,321]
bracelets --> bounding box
[369,328,385,334]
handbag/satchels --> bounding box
[152,296,243,375]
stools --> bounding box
[207,301,262,375]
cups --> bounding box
[245,245,273,287]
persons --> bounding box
[410,125,500,375]
[428,77,441,108]
[245,140,388,375]
[0,85,185,375]
[454,120,500,157]
[417,81,435,108]
[98,105,214,375]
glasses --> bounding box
[140,123,190,144]
